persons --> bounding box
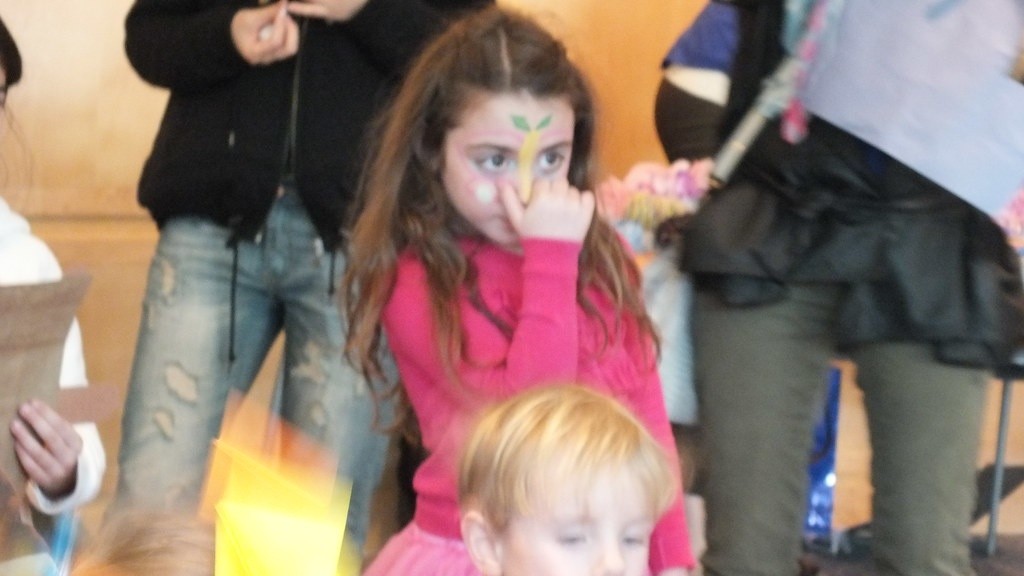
[457,384,669,576]
[2,17,109,576]
[113,0,498,576]
[655,0,1023,576]
[338,7,697,575]
